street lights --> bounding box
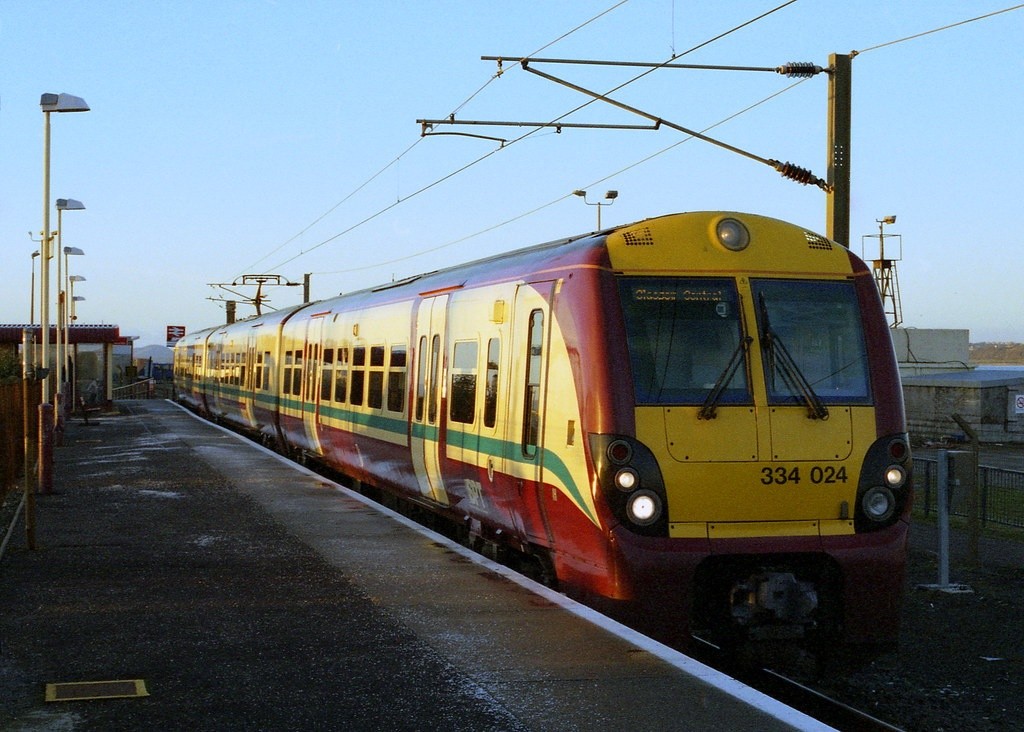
[572,189,618,231]
[29,91,92,494]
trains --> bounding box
[172,211,914,653]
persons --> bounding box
[63,355,79,420]
